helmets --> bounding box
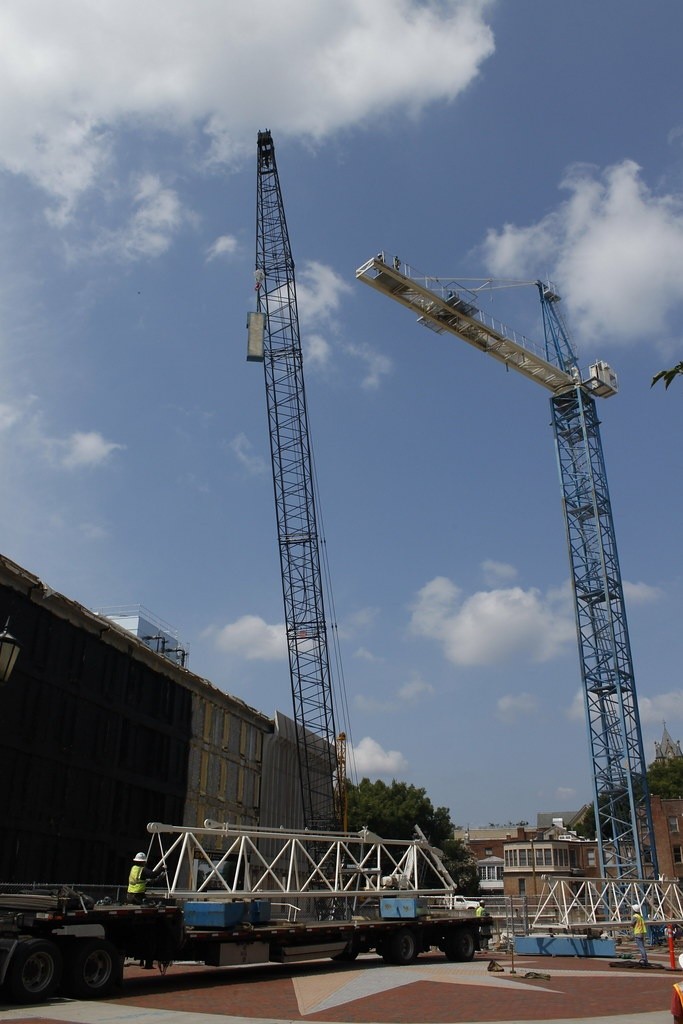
[632,904,640,912]
[133,852,147,862]
[480,900,485,904]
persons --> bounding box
[476,900,494,950]
[631,904,648,962]
[127,852,167,904]
[672,954,683,1024]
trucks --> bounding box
[361,894,480,911]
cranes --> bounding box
[248,129,353,916]
[357,250,668,955]
